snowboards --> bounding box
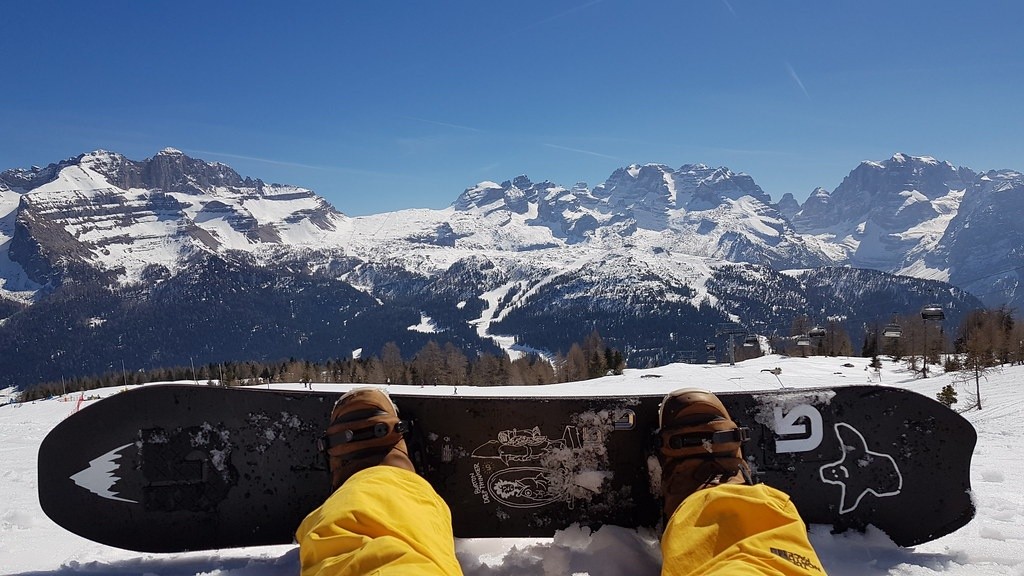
[34,373,982,555]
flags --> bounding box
[352,347,362,359]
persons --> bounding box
[454,387,457,394]
[309,379,312,390]
[421,379,424,388]
[295,386,828,576]
[435,378,437,386]
[388,377,391,386]
[305,380,307,387]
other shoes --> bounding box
[318,388,416,497]
[653,387,758,526]
